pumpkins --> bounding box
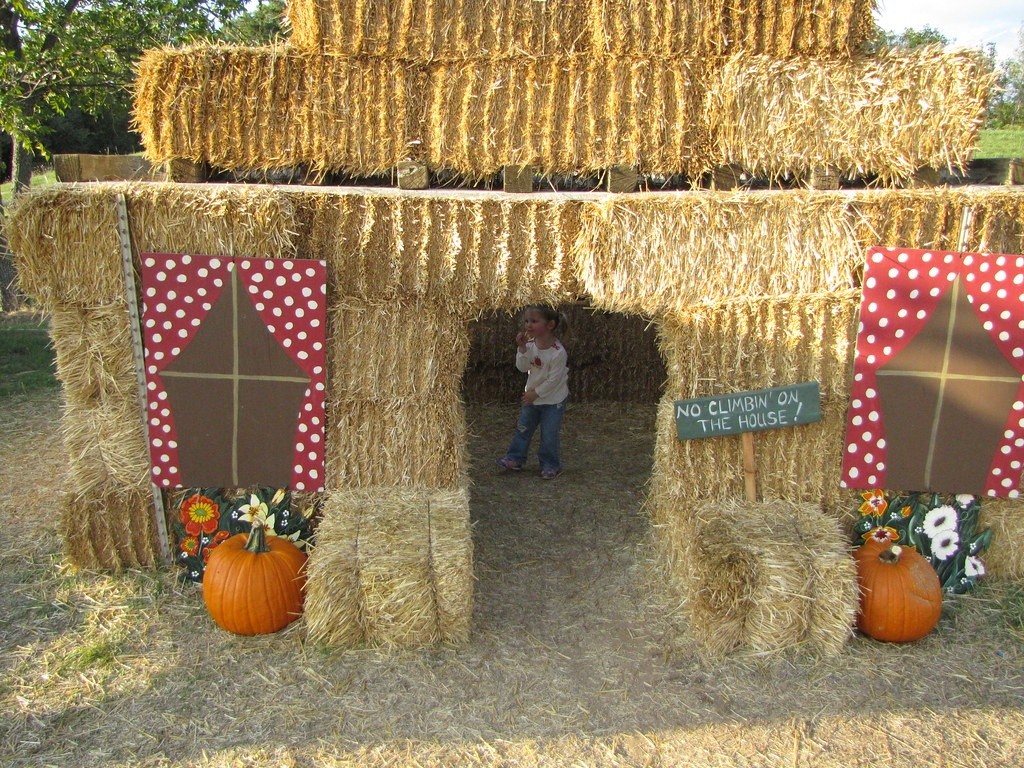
[851,542,942,643]
[203,519,307,635]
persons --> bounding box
[496,300,570,480]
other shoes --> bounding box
[495,455,522,470]
[539,462,564,480]
